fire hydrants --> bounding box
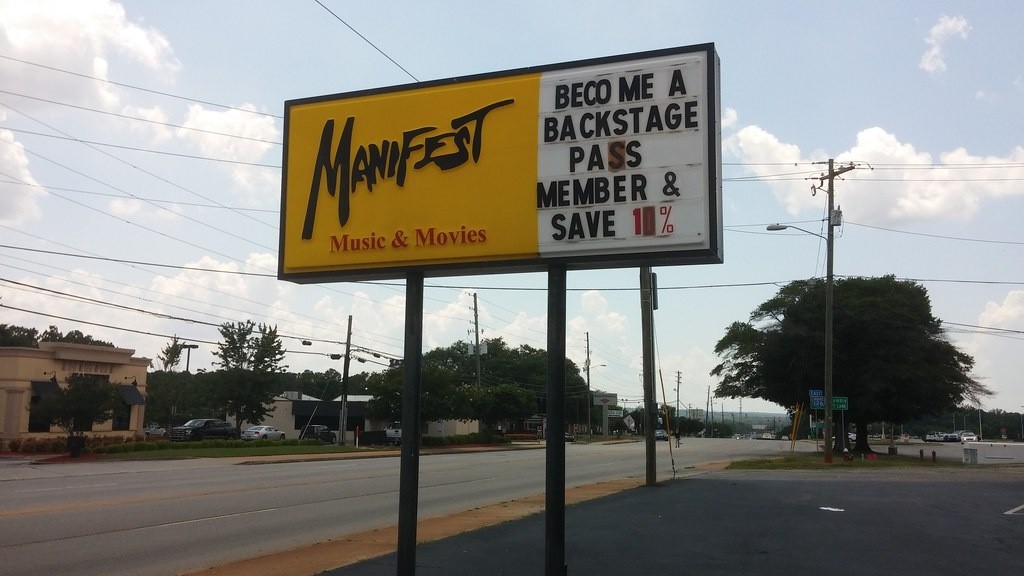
[843,447,850,461]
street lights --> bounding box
[767,223,833,465]
[586,364,607,443]
[181,344,199,372]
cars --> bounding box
[848,432,856,443]
[654,430,669,441]
[240,425,286,442]
[565,433,574,442]
[734,432,776,440]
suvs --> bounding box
[926,429,978,444]
[299,425,337,444]
[169,419,236,443]
[385,422,402,439]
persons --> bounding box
[674,429,681,448]
